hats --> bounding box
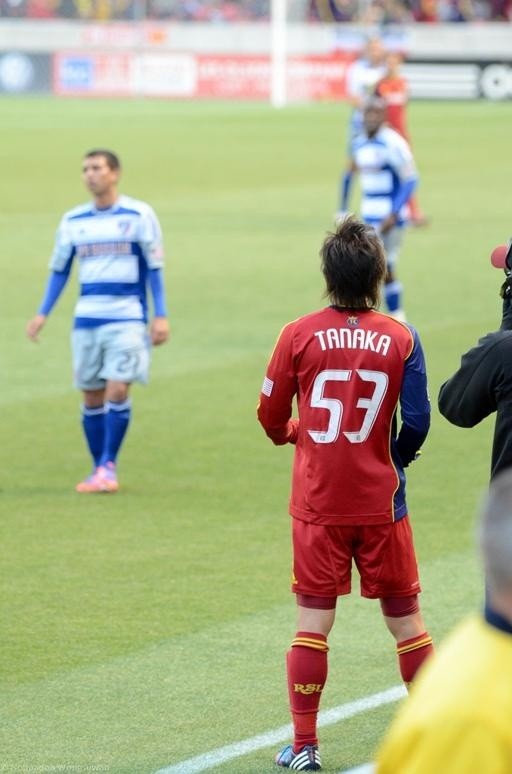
[492,246,509,268]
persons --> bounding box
[371,46,425,226]
[361,467,512,770]
[341,33,386,139]
[0,0,511,26]
[438,237,511,487]
[26,149,171,492]
[253,213,436,770]
[334,95,420,326]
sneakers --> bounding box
[276,745,321,771]
[76,460,118,493]
